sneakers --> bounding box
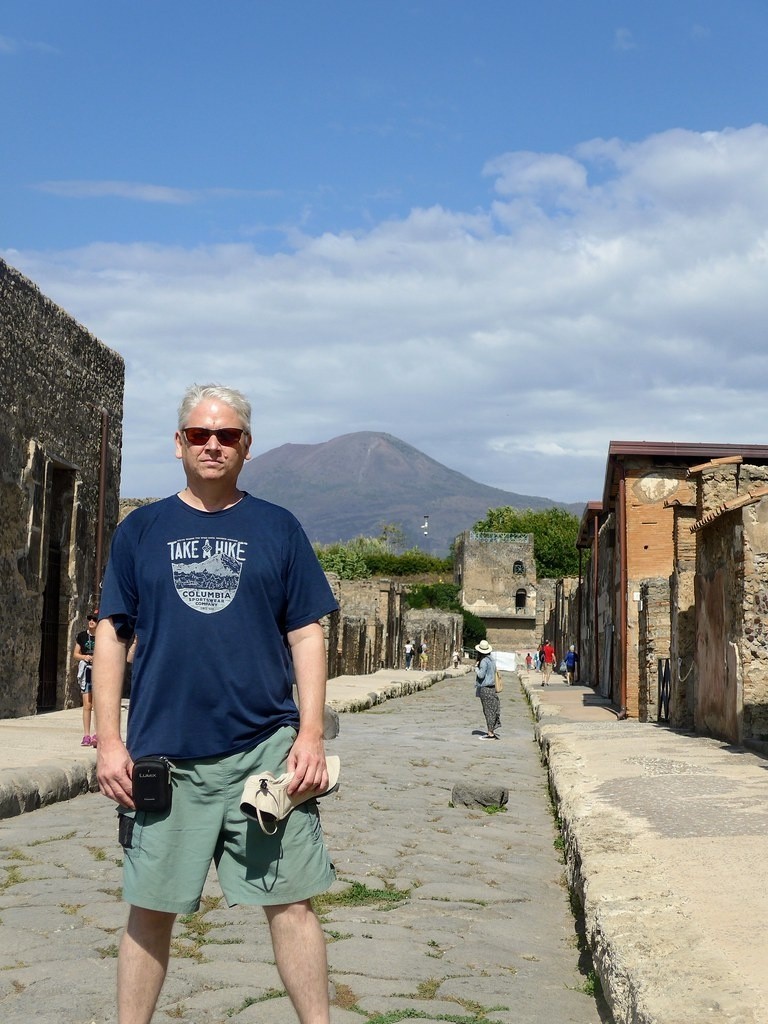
[90,734,98,748]
[81,735,92,745]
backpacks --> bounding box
[539,646,546,662]
[417,644,424,653]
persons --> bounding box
[419,639,429,672]
[404,640,414,672]
[452,649,460,668]
[91,383,342,1024]
[474,640,501,740]
[74,610,99,747]
[126,634,137,664]
[526,639,556,687]
[564,645,579,687]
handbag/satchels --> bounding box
[494,672,502,693]
[559,661,567,672]
[410,649,415,655]
[457,656,460,661]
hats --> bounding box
[475,640,492,654]
[238,754,340,834]
[90,609,100,617]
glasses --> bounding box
[86,615,98,622]
[181,427,248,447]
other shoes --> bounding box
[480,734,496,740]
[541,683,544,686]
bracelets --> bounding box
[128,647,136,657]
